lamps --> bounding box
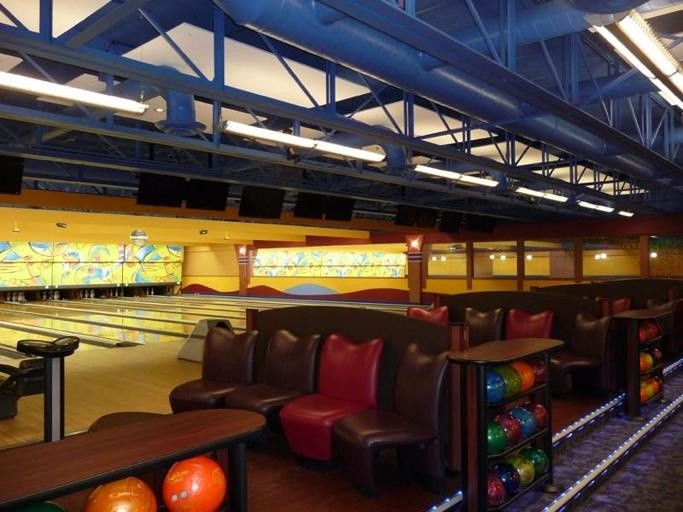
[515,186,570,205]
[594,253,608,259]
[585,7,683,112]
[577,200,615,214]
[413,164,501,187]
[221,117,386,165]
[618,209,635,218]
[0,68,150,116]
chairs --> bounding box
[168,328,258,414]
[279,334,383,461]
[551,314,609,400]
[465,307,503,347]
[225,329,320,445]
[506,307,554,338]
[407,305,449,326]
[329,344,453,497]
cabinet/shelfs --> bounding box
[0,408,267,511]
[611,309,672,418]
[446,338,566,512]
